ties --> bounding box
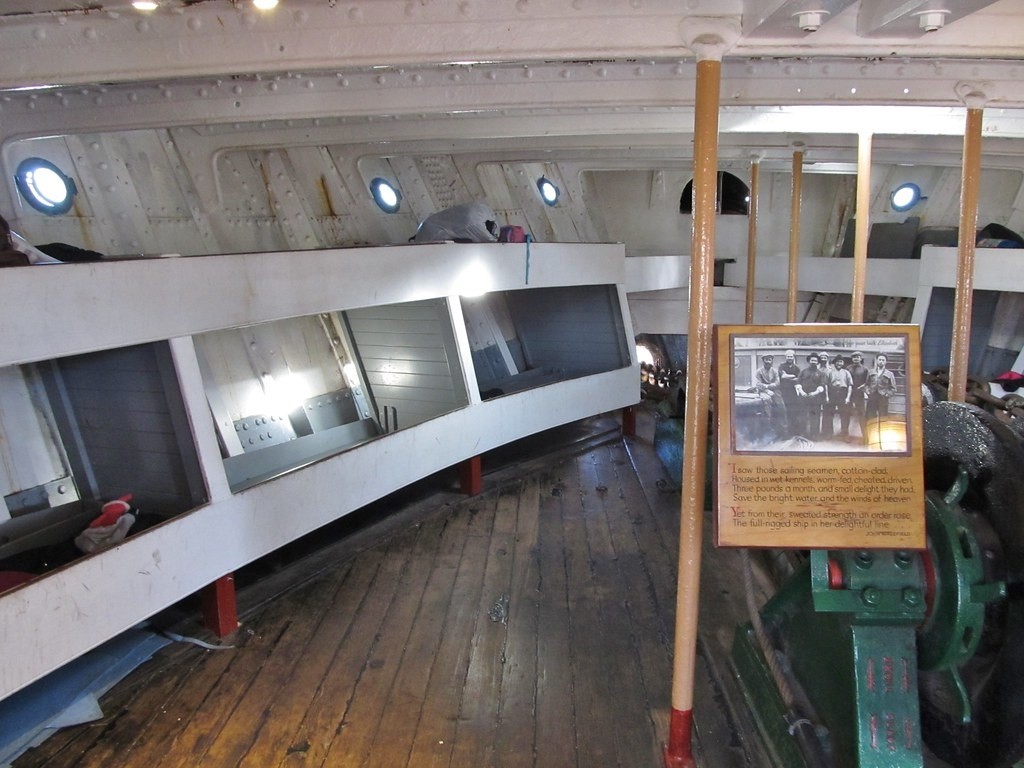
[852,366,857,384]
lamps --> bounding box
[680,171,752,218]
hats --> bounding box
[818,352,829,358]
[807,353,821,362]
[785,350,795,357]
[851,351,864,356]
[833,355,845,364]
[761,353,774,359]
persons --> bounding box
[752,348,899,443]
[0,214,106,268]
[412,202,531,246]
